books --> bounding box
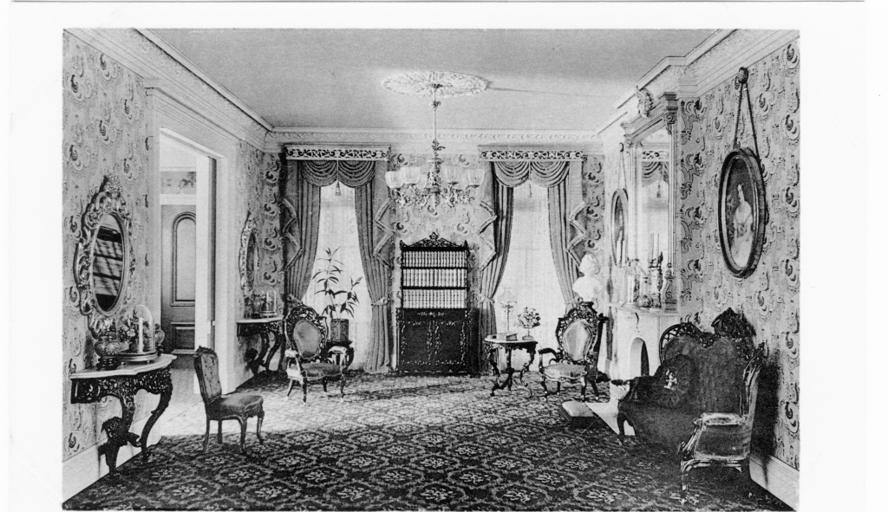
[400,248,467,310]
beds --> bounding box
[484,333,537,398]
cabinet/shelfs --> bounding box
[391,232,480,378]
[321,338,353,373]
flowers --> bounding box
[517,306,540,340]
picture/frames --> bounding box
[717,147,767,279]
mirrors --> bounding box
[624,122,676,267]
[69,172,139,337]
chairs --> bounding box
[283,303,346,402]
[191,343,266,452]
[537,308,605,400]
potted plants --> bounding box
[314,247,363,340]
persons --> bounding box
[569,254,603,303]
[728,184,754,268]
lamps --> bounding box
[385,83,484,210]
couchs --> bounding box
[612,305,770,500]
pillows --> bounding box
[650,352,698,408]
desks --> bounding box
[71,353,181,479]
[237,315,284,376]
